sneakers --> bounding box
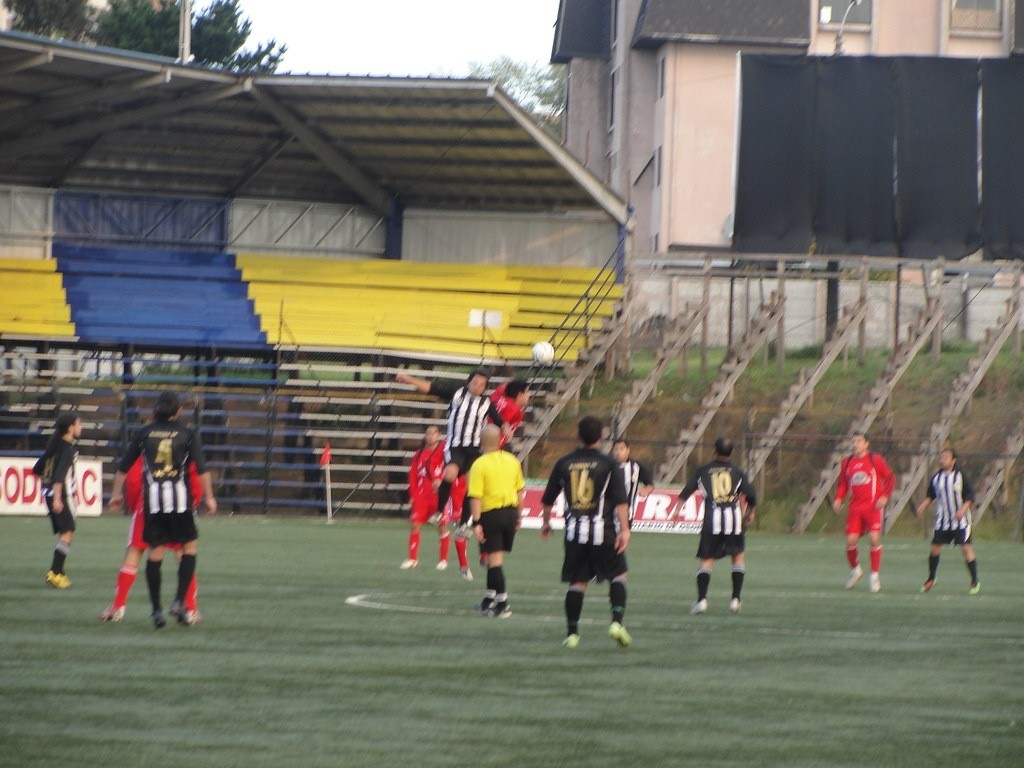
[609,622,630,647]
[562,634,577,648]
[475,597,512,619]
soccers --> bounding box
[532,340,556,366]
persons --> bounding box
[541,417,634,648]
[832,431,896,593]
[32,412,83,589]
[99,390,218,628]
[672,436,756,615]
[917,447,980,595]
[467,423,526,619]
[393,370,530,539]
[611,440,654,530]
[399,426,474,583]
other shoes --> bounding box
[969,582,980,595]
[870,573,880,592]
[690,598,707,615]
[45,571,72,589]
[186,609,198,625]
[169,599,189,626]
[846,565,864,590]
[103,605,125,622]
[436,560,447,570]
[400,559,417,569]
[729,598,739,614]
[463,568,473,582]
[920,577,937,594]
[152,608,167,629]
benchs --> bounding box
[0,243,626,512]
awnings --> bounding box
[0,51,637,235]
